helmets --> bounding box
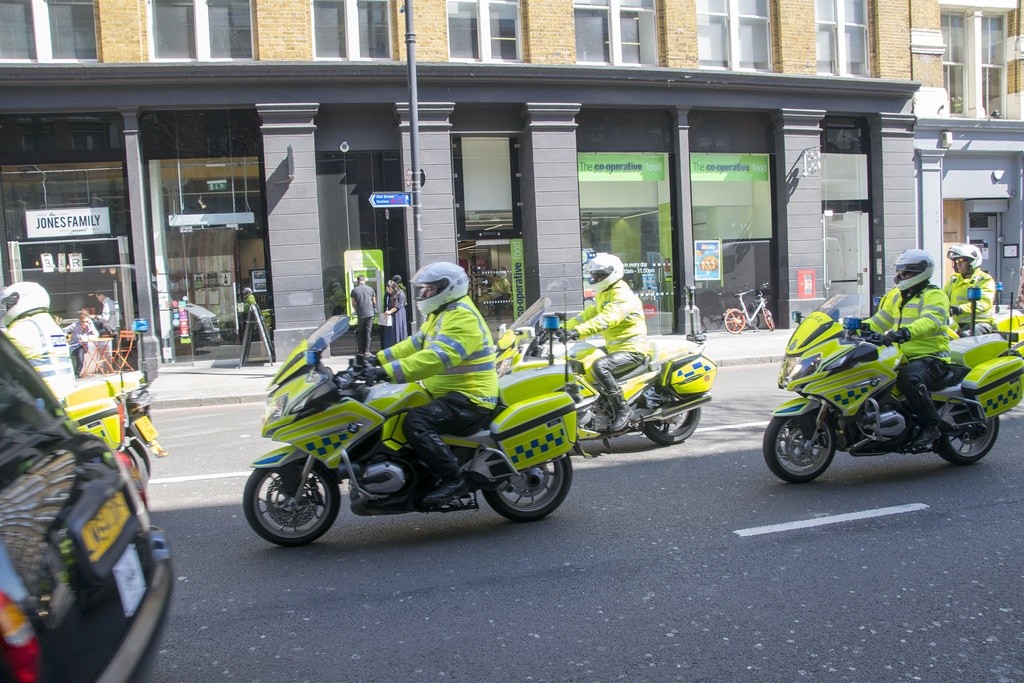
[586,252,625,293]
[946,245,983,274]
[893,249,935,290]
[410,260,469,314]
[242,288,252,298]
[1,281,52,327]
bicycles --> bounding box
[701,283,760,330]
[724,282,775,334]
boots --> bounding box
[609,390,635,432]
[408,430,471,507]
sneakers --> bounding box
[913,426,941,444]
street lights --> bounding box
[340,141,350,251]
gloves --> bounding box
[367,355,380,366]
[883,325,911,348]
[949,304,962,317]
[559,330,579,345]
[365,365,386,387]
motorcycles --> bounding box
[763,287,1024,484]
[873,282,1024,360]
[494,297,717,460]
[242,315,577,547]
[60,318,168,490]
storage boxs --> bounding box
[960,356,1024,420]
[489,392,577,471]
[63,398,125,451]
[659,353,717,401]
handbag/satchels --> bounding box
[378,312,393,326]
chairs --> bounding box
[103,331,135,376]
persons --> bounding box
[0,282,77,402]
[95,292,117,338]
[855,248,952,446]
[559,254,648,433]
[497,272,510,294]
[941,244,996,338]
[61,313,99,378]
[393,275,406,292]
[353,261,499,504]
[383,280,408,349]
[242,287,256,312]
[327,282,345,303]
[350,275,376,357]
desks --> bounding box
[78,337,117,377]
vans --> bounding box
[1,264,221,362]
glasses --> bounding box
[955,257,968,264]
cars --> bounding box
[0,330,172,683]
